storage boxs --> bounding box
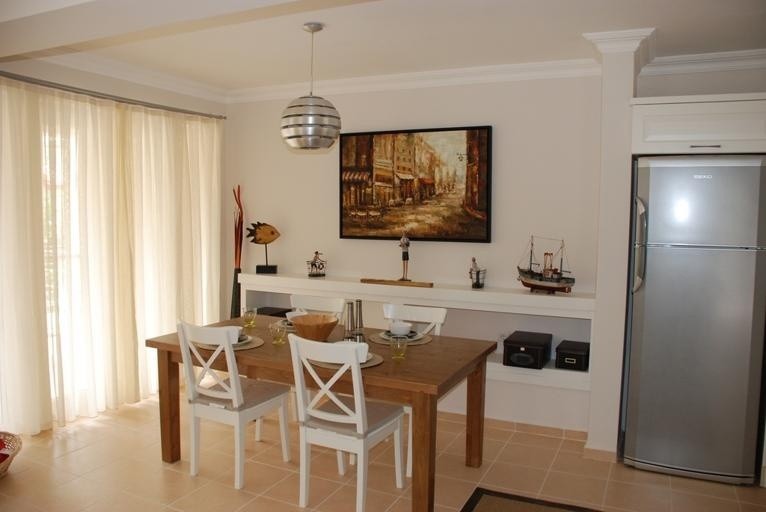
[502,330,552,369]
[555,339,590,371]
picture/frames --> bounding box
[339,126,491,243]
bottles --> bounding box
[345,299,363,331]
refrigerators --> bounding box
[622,156,765,490]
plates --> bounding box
[230,336,252,346]
[384,330,417,337]
[279,319,295,328]
[380,332,422,342]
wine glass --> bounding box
[269,322,287,345]
[241,306,257,328]
[392,336,406,359]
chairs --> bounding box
[174,317,291,490]
[350,304,448,477]
[286,333,406,512]
[289,294,347,423]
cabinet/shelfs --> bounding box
[237,273,596,392]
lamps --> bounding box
[280,22,341,153]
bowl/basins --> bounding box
[390,323,412,334]
[236,326,243,336]
[285,311,306,321]
[289,314,339,340]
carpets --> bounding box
[459,487,606,512]
